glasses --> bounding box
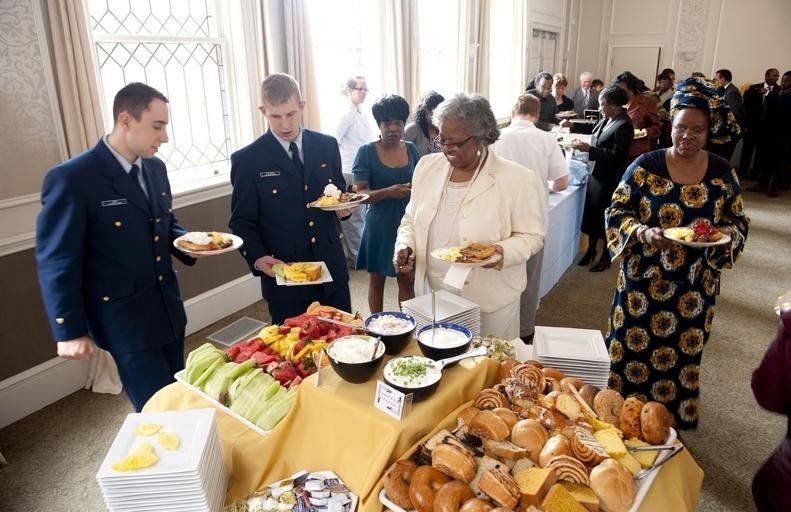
[350,86,368,94]
[671,121,709,136]
[431,133,476,149]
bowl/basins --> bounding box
[363,311,415,355]
[328,335,386,382]
[384,355,441,400]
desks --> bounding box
[139,302,704,512]
[538,176,590,299]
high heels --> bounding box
[575,246,598,268]
[588,246,612,272]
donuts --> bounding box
[407,465,512,512]
[383,458,419,512]
[526,360,671,443]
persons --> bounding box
[35,83,208,412]
[494,66,790,271]
[751,313,791,512]
[352,94,420,314]
[393,93,547,340]
[330,74,375,265]
[407,92,444,159]
[226,74,359,325]
[603,76,751,431]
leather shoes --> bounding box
[742,178,787,200]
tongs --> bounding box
[629,443,685,480]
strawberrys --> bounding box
[225,336,318,390]
[277,311,356,343]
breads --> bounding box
[176,239,221,252]
[302,264,323,281]
[421,359,655,511]
[461,242,495,260]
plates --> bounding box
[531,325,613,392]
[173,231,244,256]
[555,110,577,118]
[430,248,503,267]
[309,195,369,211]
[399,288,481,338]
[275,261,333,288]
[635,133,647,139]
[664,225,731,252]
[99,408,228,511]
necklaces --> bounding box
[434,146,486,245]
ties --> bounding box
[286,139,306,178]
[584,92,590,105]
[125,161,150,208]
